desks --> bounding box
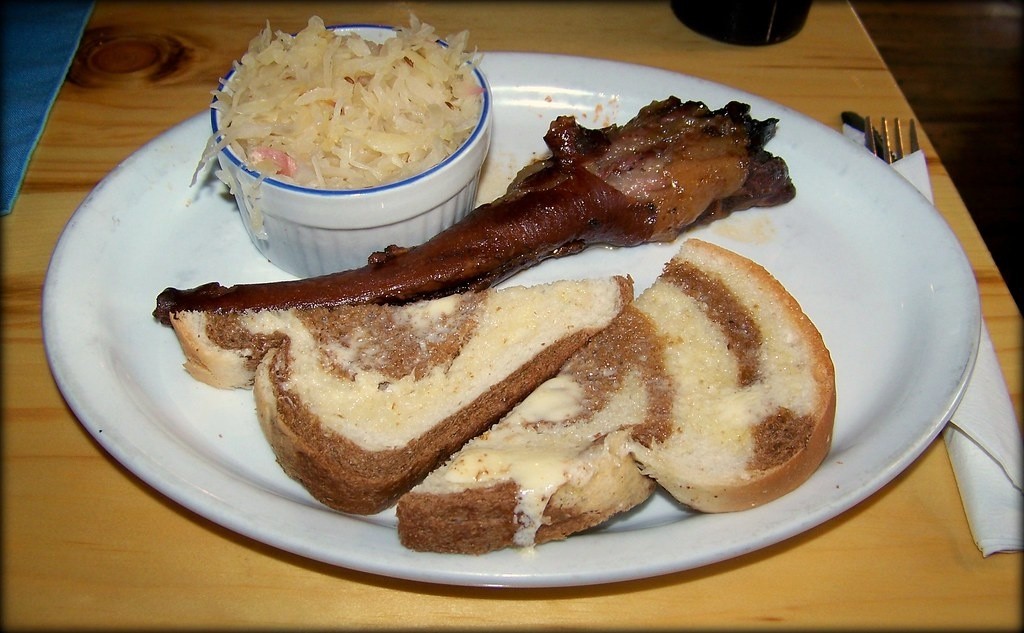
[0,0,1024,633]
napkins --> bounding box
[842,122,1024,558]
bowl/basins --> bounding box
[212,24,490,278]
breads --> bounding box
[170,273,635,515]
[392,237,838,554]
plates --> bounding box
[41,53,981,587]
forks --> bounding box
[865,116,919,164]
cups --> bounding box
[667,0,812,46]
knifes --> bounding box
[840,111,892,164]
